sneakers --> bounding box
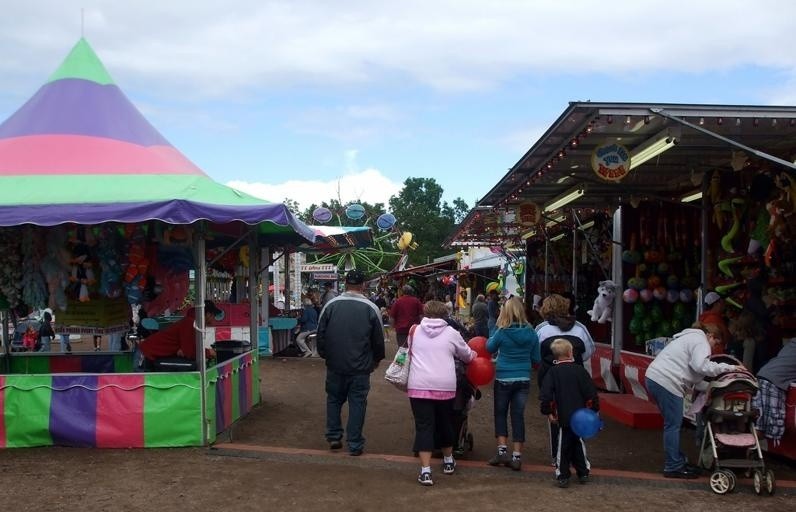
[349,449,363,456]
[506,461,521,470]
[444,457,456,473]
[331,443,342,451]
[581,477,587,483]
[559,479,568,487]
[417,472,433,485]
[488,452,507,465]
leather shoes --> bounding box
[664,463,702,478]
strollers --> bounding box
[694,354,775,497]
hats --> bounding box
[347,268,366,285]
[204,300,221,315]
[704,292,720,305]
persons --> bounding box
[33,312,55,353]
[486,299,542,470]
[137,300,222,370]
[22,324,39,353]
[407,299,478,486]
[280,274,503,358]
[316,269,386,455]
[694,291,732,449]
[535,294,597,470]
[540,338,603,487]
[751,335,796,447]
[645,321,748,477]
[500,289,580,328]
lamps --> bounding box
[541,182,587,213]
[628,127,681,172]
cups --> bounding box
[92,335,101,352]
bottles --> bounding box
[60,333,71,354]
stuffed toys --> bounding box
[619,174,796,353]
[587,279,618,324]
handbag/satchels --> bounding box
[384,347,410,392]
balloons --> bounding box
[467,358,496,385]
[468,335,493,361]
[571,408,602,439]
